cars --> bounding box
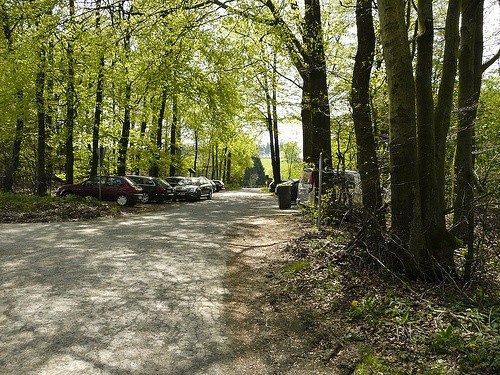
[274,180,300,200]
[170,176,213,200]
[209,181,217,193]
[164,177,187,187]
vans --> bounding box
[296,169,389,210]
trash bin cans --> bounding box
[275,183,292,209]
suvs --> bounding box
[212,180,225,192]
[54,175,144,207]
[268,181,287,192]
[122,175,175,202]
